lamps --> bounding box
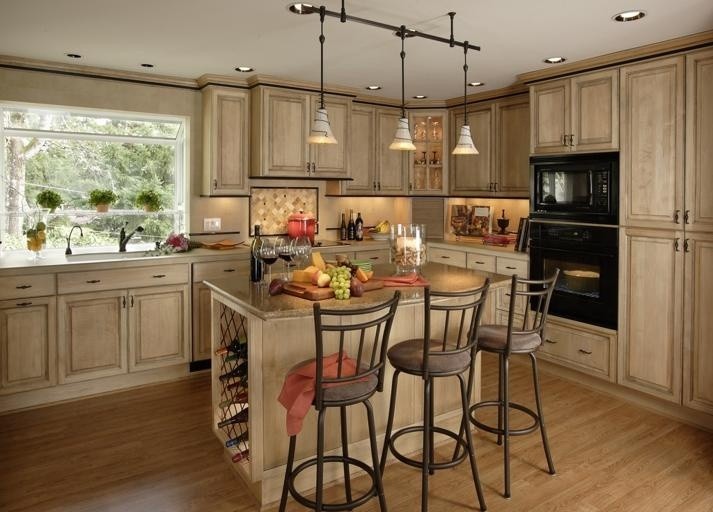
[305,5,338,143]
[388,24,418,152]
[451,39,481,155]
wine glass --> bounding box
[252,237,312,289]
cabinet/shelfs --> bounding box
[494,254,617,384]
[618,47,713,235]
[425,242,497,328]
[616,228,713,433]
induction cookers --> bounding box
[311,239,350,247]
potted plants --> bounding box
[87,188,119,212]
[37,189,62,213]
[134,191,165,213]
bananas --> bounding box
[376,219,391,232]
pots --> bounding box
[287,211,319,246]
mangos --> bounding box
[368,227,378,233]
[31,231,45,251]
[316,273,331,288]
[367,225,376,228]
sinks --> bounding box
[65,250,182,263]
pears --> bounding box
[311,269,323,286]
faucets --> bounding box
[118,225,144,252]
[64,225,84,254]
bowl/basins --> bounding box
[368,231,390,240]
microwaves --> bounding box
[530,152,619,225]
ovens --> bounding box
[528,219,619,330]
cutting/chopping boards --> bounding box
[283,278,384,301]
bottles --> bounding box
[348,208,355,240]
[355,213,363,241]
[215,337,248,463]
[250,225,261,282]
[341,214,346,241]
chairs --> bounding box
[375,279,490,511]
[279,289,401,511]
[452,267,561,501]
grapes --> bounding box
[323,265,354,301]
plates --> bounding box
[201,239,244,248]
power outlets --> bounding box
[204,218,221,233]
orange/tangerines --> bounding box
[26,222,46,238]
